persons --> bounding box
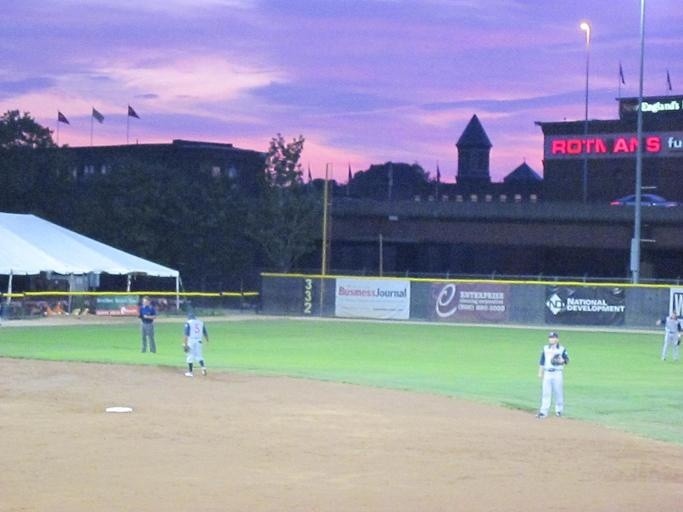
[138,296,157,353]
[536,331,569,419]
[182,313,210,377]
[655,312,681,361]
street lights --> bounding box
[578,20,590,203]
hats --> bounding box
[188,313,195,319]
[548,332,558,338]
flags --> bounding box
[667,72,671,90]
[619,65,625,85]
[128,106,139,119]
[58,112,69,124]
[93,108,104,124]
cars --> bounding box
[610,190,677,209]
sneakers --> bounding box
[556,411,561,417]
[185,371,193,377]
[536,413,547,418]
[202,367,207,376]
